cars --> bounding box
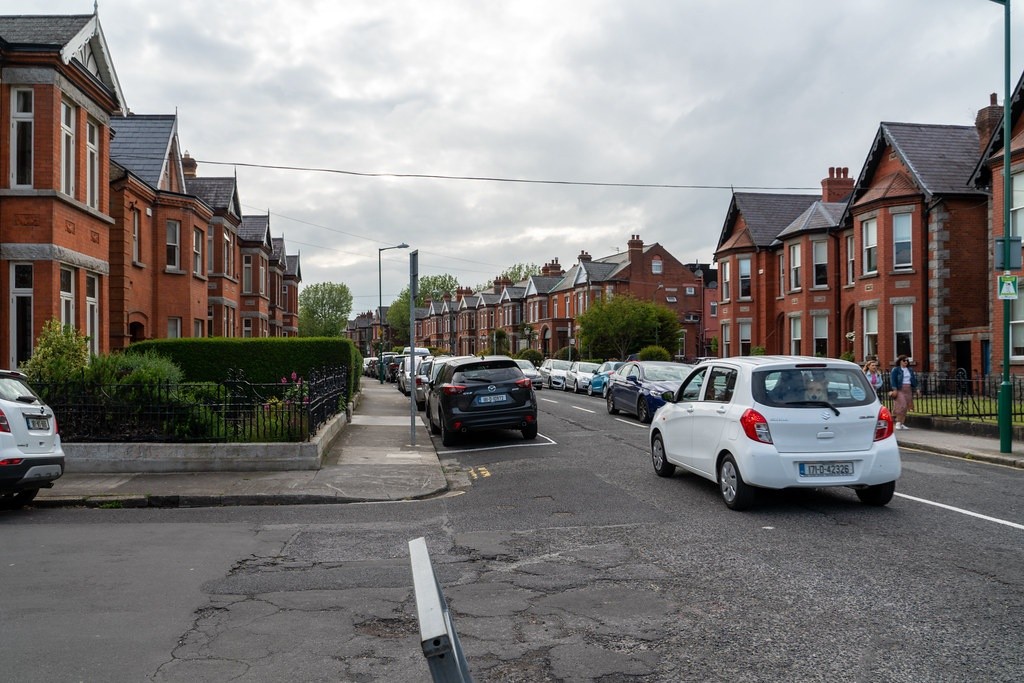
[649,355,902,511]
[428,355,539,447]
[363,347,627,416]
[1,369,67,506]
[606,361,704,424]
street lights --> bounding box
[378,243,410,384]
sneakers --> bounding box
[900,424,910,430]
[895,422,901,430]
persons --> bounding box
[863,361,883,394]
[889,356,920,430]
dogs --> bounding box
[804,377,835,405]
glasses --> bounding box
[903,359,908,362]
